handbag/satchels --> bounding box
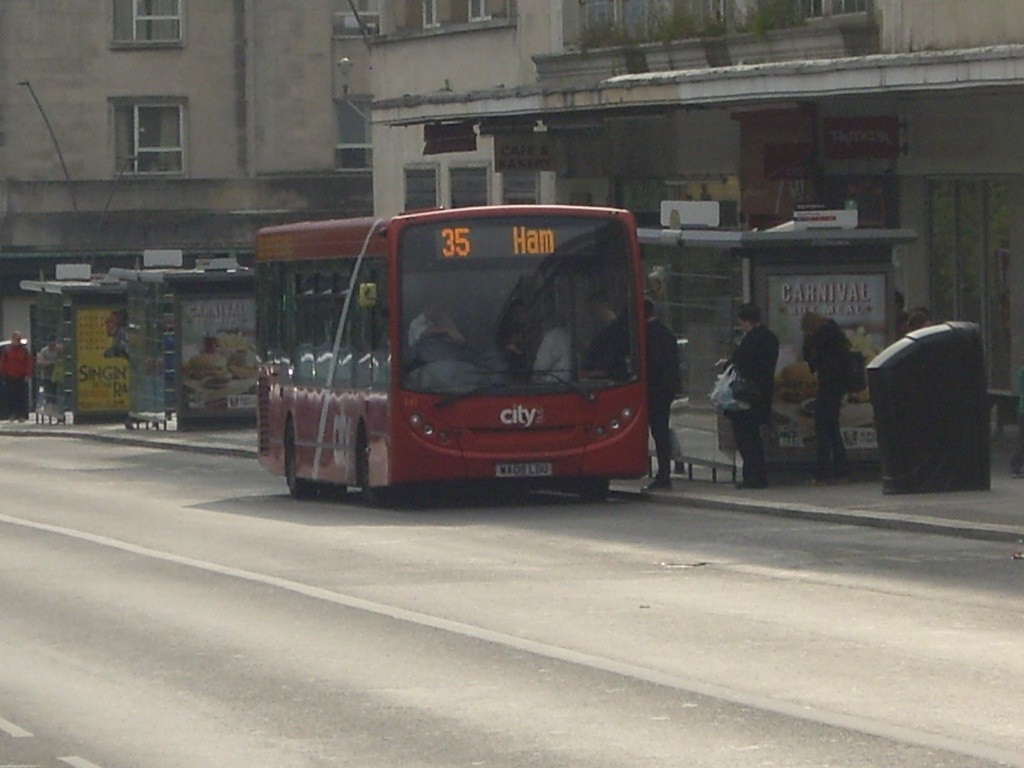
[844,350,866,392]
[50,363,64,383]
[709,365,753,414]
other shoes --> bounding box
[641,477,673,492]
[735,474,768,490]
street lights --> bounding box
[14,79,88,263]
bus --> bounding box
[253,203,650,510]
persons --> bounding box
[499,301,573,382]
[642,300,679,492]
[895,291,930,341]
[724,306,779,490]
[0,331,34,422]
[39,334,63,404]
[408,296,465,351]
[800,313,852,483]
[589,295,628,378]
